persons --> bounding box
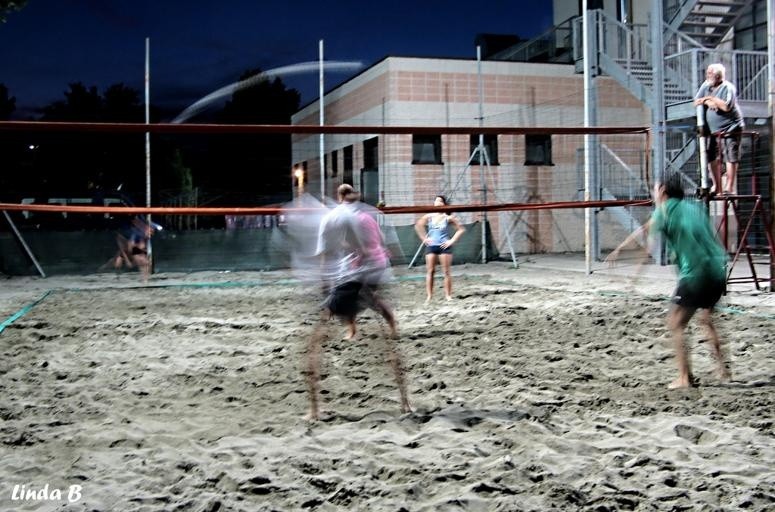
[413,194,467,309]
[300,181,416,427]
[112,214,161,282]
[599,172,735,392]
[328,207,402,345]
[690,60,745,197]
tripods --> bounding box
[497,166,578,257]
[408,44,521,267]
